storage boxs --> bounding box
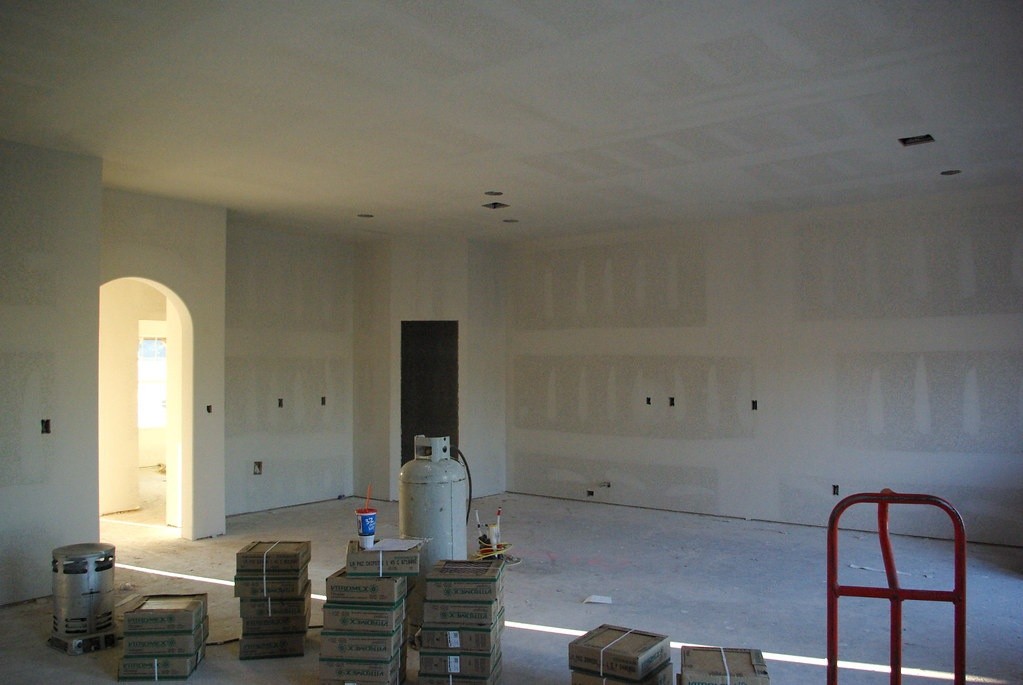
[117,592,210,680]
[318,540,507,685]
[234,540,312,659]
[47,628,118,655]
[569,623,770,684]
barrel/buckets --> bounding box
[51,544,114,637]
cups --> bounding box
[355,509,378,548]
